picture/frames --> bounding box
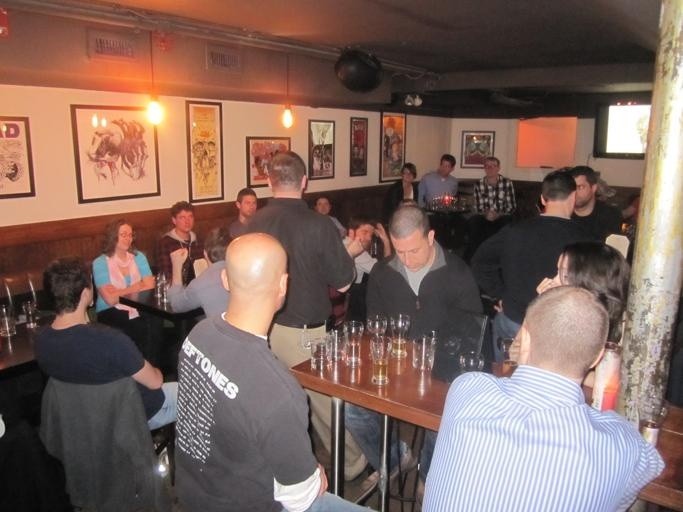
[308,119,336,181]
[350,116,368,176]
[185,99,225,204]
[460,130,498,170]
[380,107,408,182]
[1,116,37,199]
[69,103,162,205]
[245,136,292,188]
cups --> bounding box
[370,336,393,385]
[412,335,436,370]
[638,405,668,448]
[22,301,38,328]
[343,321,364,368]
[326,330,344,361]
[460,351,485,372]
[305,337,333,366]
[431,193,467,211]
[0,305,16,337]
[390,314,411,358]
[367,314,387,359]
[153,272,172,304]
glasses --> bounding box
[120,233,133,238]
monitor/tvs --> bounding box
[592,91,652,160]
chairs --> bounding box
[374,311,490,512]
[0,268,44,307]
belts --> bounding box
[291,322,326,329]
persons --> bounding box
[31,255,179,431]
[92,217,163,370]
[314,154,683,411]
[421,286,666,512]
[174,232,377,512]
[157,201,207,352]
[343,204,484,504]
[169,229,236,354]
[226,187,258,239]
[243,151,370,482]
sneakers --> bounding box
[385,443,417,480]
[344,453,368,480]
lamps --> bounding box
[143,30,164,128]
[282,52,294,129]
